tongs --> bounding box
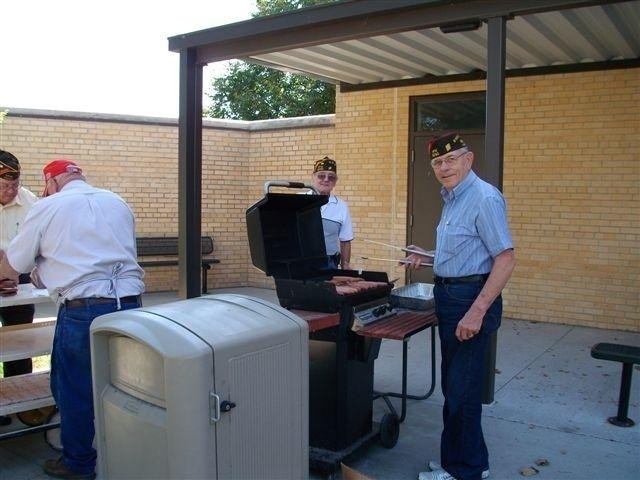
[360,237,436,268]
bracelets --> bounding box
[341,260,350,268]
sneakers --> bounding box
[17,409,45,426]
[45,459,95,480]
[419,469,482,480]
[428,461,489,479]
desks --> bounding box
[354,311,439,423]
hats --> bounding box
[0,149,21,180]
[313,156,336,174]
[429,132,467,160]
[43,160,82,196]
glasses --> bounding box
[314,174,336,181]
[431,151,467,168]
[0,181,22,191]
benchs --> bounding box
[137,237,220,294]
[591,342,640,427]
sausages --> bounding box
[325,276,387,296]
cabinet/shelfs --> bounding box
[0,283,60,437]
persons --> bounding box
[0,150,38,377]
[0,159,147,480]
[291,157,356,271]
[396,131,518,480]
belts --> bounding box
[67,297,137,307]
[434,274,488,285]
